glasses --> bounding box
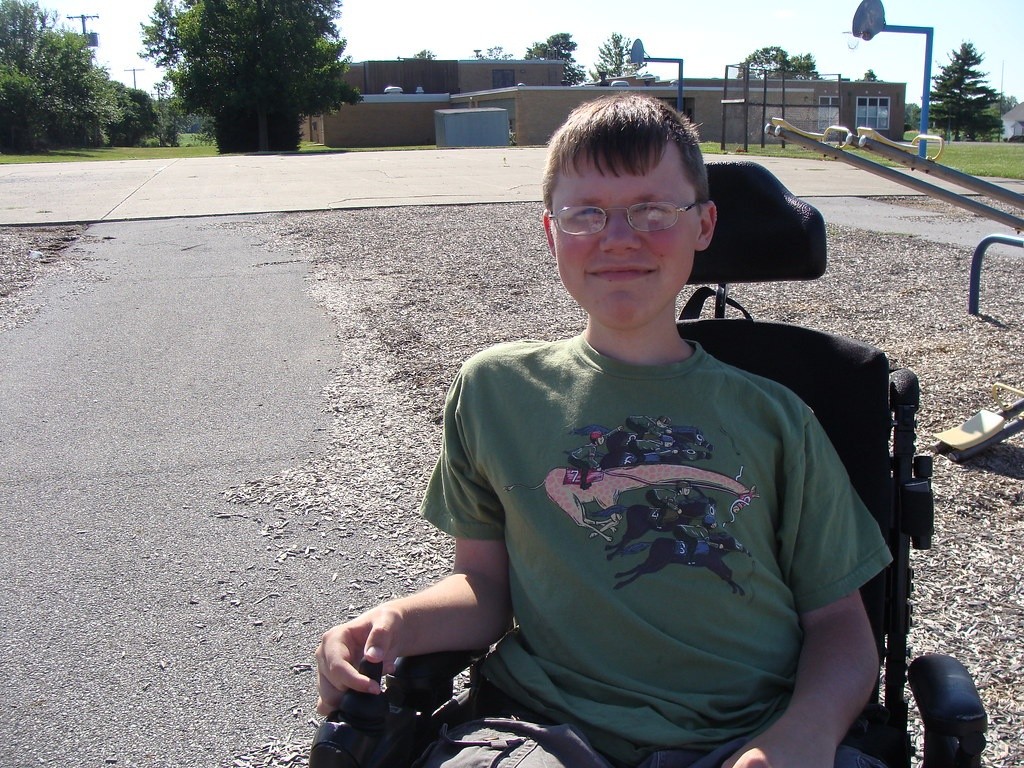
[547,201,698,236]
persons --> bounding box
[316,94,894,768]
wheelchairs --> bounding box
[306,161,990,767]
